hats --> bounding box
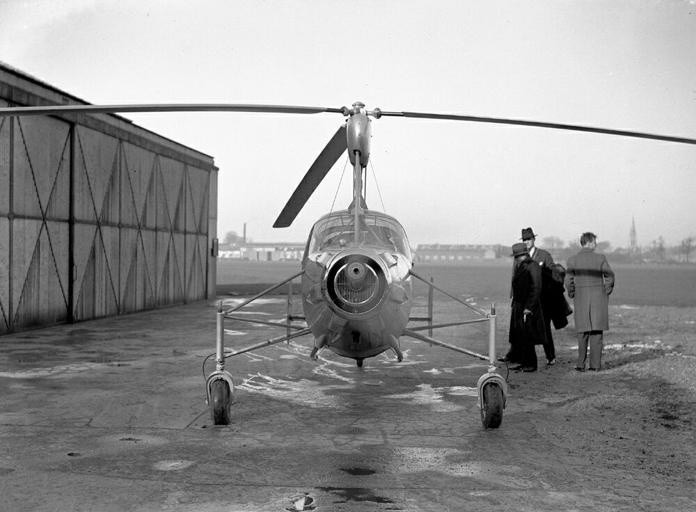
[510,243,529,256]
[519,228,536,240]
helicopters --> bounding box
[0,99,696,433]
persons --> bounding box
[508,242,543,373]
[565,232,616,374]
[498,227,561,365]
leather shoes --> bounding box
[576,367,599,372]
[498,354,536,373]
[547,359,555,365]
